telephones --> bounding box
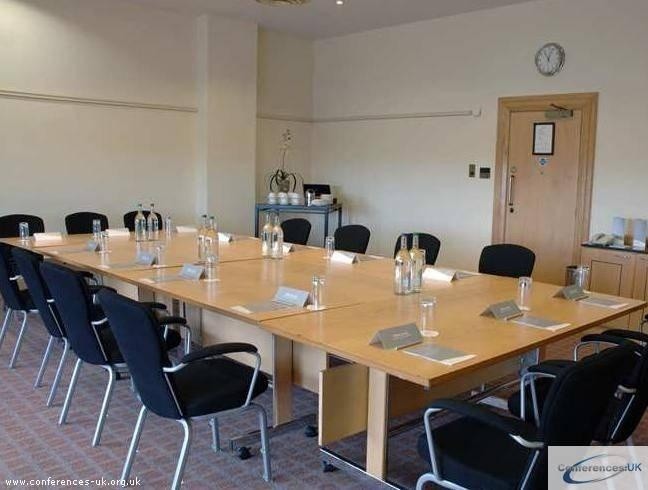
[592,232,615,246]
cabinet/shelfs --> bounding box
[580,244,648,334]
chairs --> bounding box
[0,214,44,238]
[0,245,39,371]
[505,317,648,489]
[123,210,163,232]
[64,212,109,235]
[414,343,635,489]
[10,247,120,407]
[97,289,271,490]
[276,217,535,281]
[39,261,192,446]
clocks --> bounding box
[533,42,567,77]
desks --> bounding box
[0,223,644,488]
[253,201,341,249]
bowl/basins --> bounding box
[312,199,326,206]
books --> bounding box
[403,342,478,366]
[511,314,575,333]
[577,297,629,309]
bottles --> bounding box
[205,215,218,261]
[624,209,634,247]
[395,236,412,295]
[261,210,272,259]
[147,203,159,241]
[135,202,146,242]
[271,213,284,258]
[197,214,208,262]
[410,235,425,295]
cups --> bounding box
[321,194,333,205]
[565,265,589,293]
[99,232,111,254]
[309,274,328,312]
[324,237,336,257]
[205,256,219,281]
[418,295,439,338]
[265,192,305,206]
[92,220,102,235]
[163,218,171,234]
[516,277,533,311]
[19,222,29,240]
[305,191,316,207]
[153,244,164,269]
